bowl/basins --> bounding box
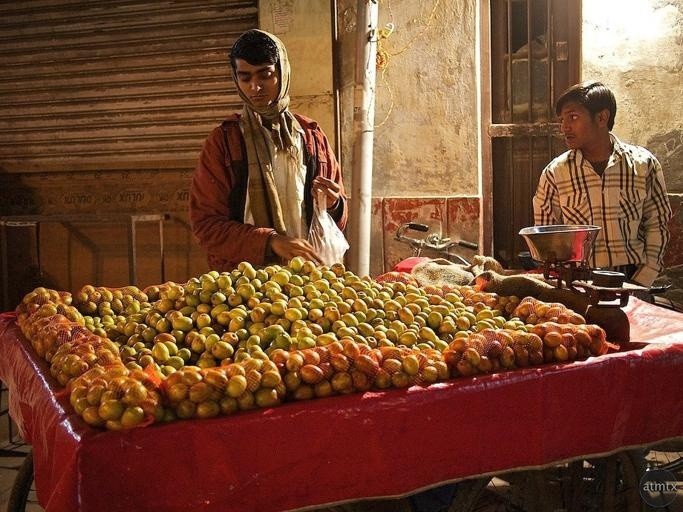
[517,225,602,262]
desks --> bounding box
[0,278,683,512]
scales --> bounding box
[518,224,672,308]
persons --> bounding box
[187,28,349,275]
[531,81,674,305]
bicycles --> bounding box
[393,223,538,270]
